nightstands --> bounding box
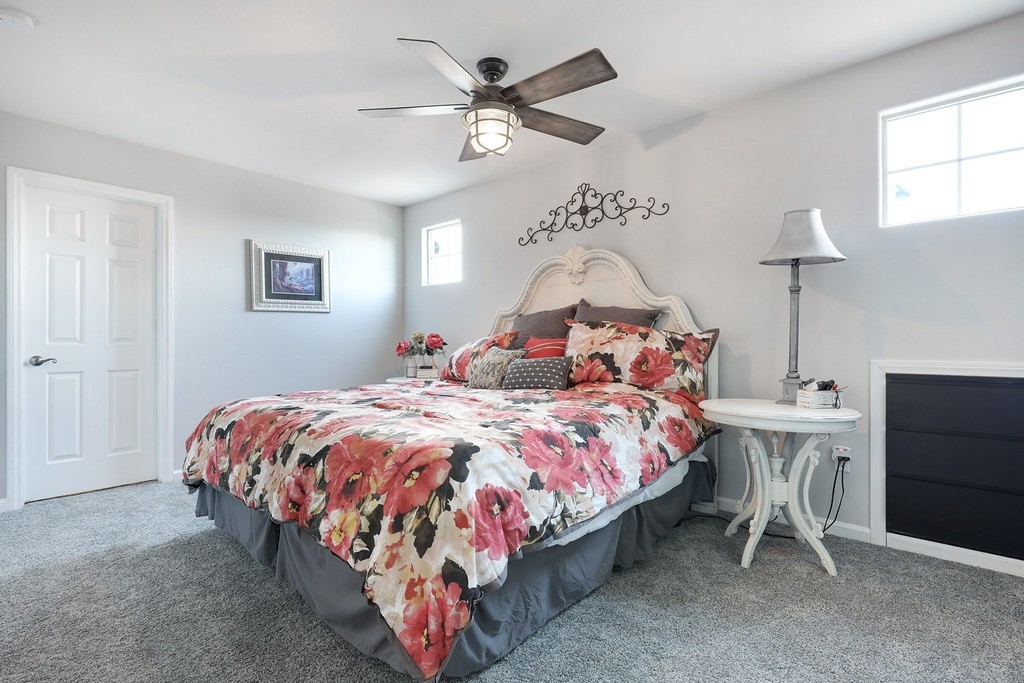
[385,376,434,384]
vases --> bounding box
[418,354,426,369]
[406,355,417,377]
[429,355,438,378]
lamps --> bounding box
[461,100,522,157]
[759,208,847,404]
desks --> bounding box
[701,397,863,575]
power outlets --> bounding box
[831,445,851,472]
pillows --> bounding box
[566,298,664,339]
[439,327,519,382]
[502,356,574,389]
[507,301,579,350]
[563,318,720,412]
[466,345,528,390]
[522,336,567,358]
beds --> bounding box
[181,244,719,683]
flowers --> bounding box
[397,333,445,356]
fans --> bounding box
[357,38,617,162]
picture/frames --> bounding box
[248,239,331,313]
